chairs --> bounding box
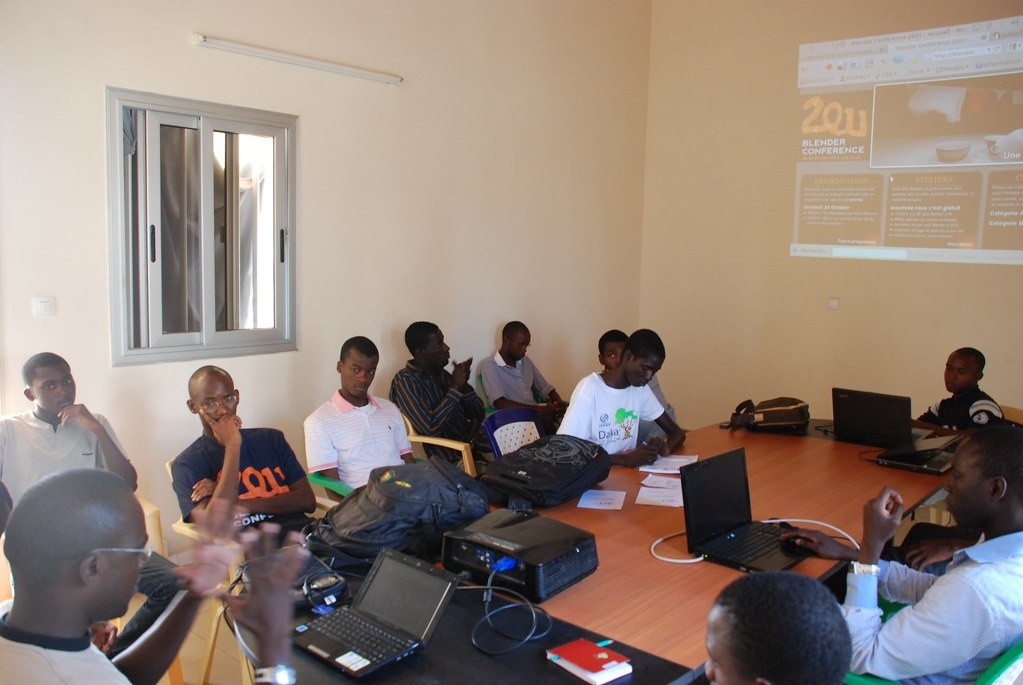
[0,375,546,685]
[843,593,1023,685]
[930,406,1023,527]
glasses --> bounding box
[191,393,237,412]
[89,545,149,562]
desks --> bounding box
[238,419,967,685]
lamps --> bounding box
[192,34,405,86]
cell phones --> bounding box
[720,422,730,429]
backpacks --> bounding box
[296,454,490,579]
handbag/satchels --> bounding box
[727,396,811,436]
[473,434,612,511]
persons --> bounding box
[171,365,316,537]
[0,352,188,660]
[481,321,569,435]
[555,329,686,468]
[303,336,416,502]
[389,321,495,478]
[598,330,678,446]
[917,347,1005,431]
[705,570,853,685]
[779,425,1023,685]
[0,469,310,685]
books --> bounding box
[546,638,633,685]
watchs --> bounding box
[254,665,298,685]
[847,561,880,576]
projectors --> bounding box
[441,506,599,605]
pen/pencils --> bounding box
[641,440,661,458]
[549,640,614,661]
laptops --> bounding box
[290,546,462,681]
[680,447,813,573]
[830,387,934,448]
[875,433,965,475]
